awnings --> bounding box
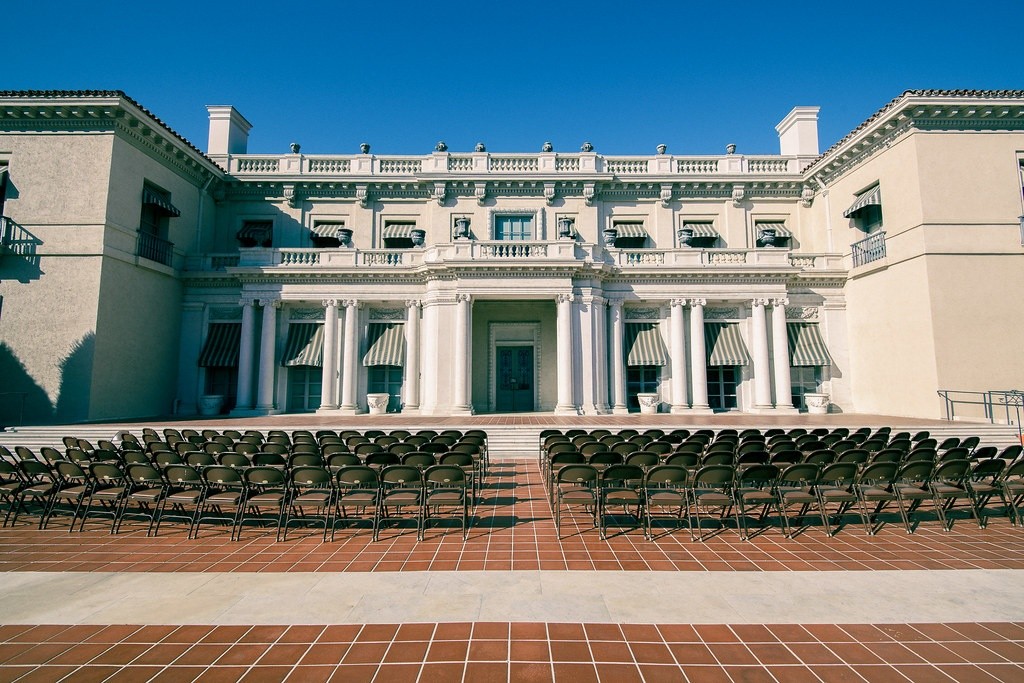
[362,323,405,368]
[0,164,9,176]
[280,322,325,366]
[235,222,272,240]
[310,223,344,239]
[613,223,649,238]
[196,323,241,367]
[786,322,832,367]
[142,180,182,219]
[704,322,749,366]
[685,222,719,238]
[844,184,881,219]
[625,323,666,366]
[382,223,414,239]
[755,221,792,242]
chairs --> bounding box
[542,421,1023,540]
[1,427,494,540]
